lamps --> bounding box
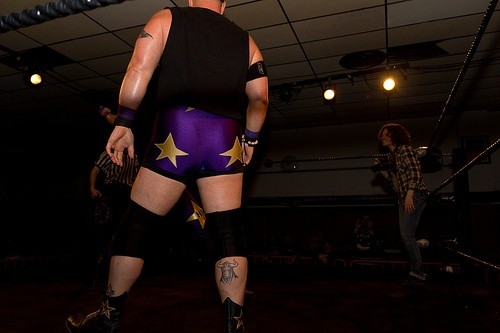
[278,82,293,103]
[323,76,336,104]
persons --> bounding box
[372,123,430,285]
[65,1,269,333]
[90,150,138,245]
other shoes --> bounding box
[66,290,127,333]
[410,270,428,281]
[200,296,246,332]
